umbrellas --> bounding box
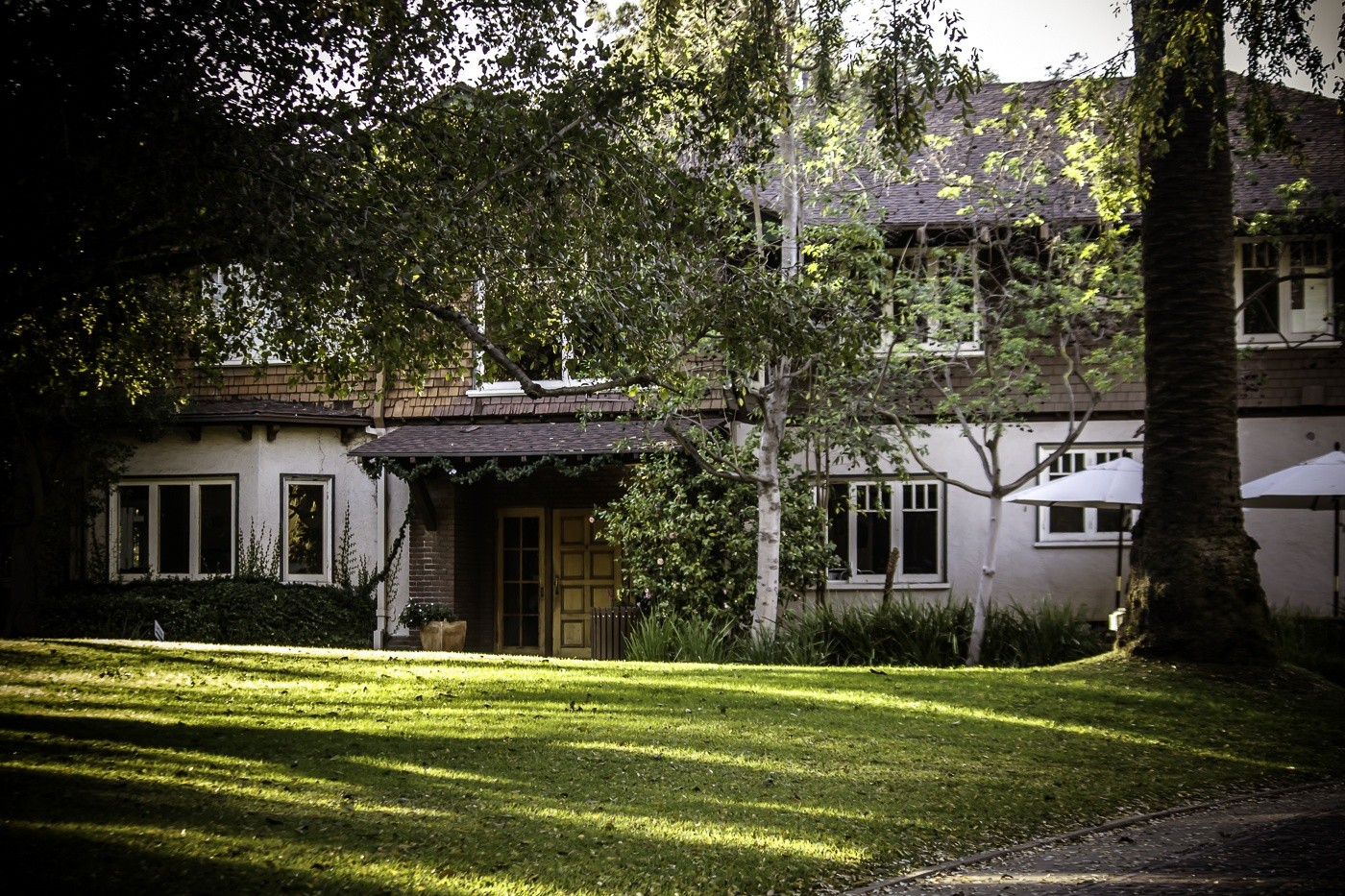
[1001,457,1144,607]
[1240,450,1345,622]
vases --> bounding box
[421,620,467,651]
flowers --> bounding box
[398,598,457,628]
